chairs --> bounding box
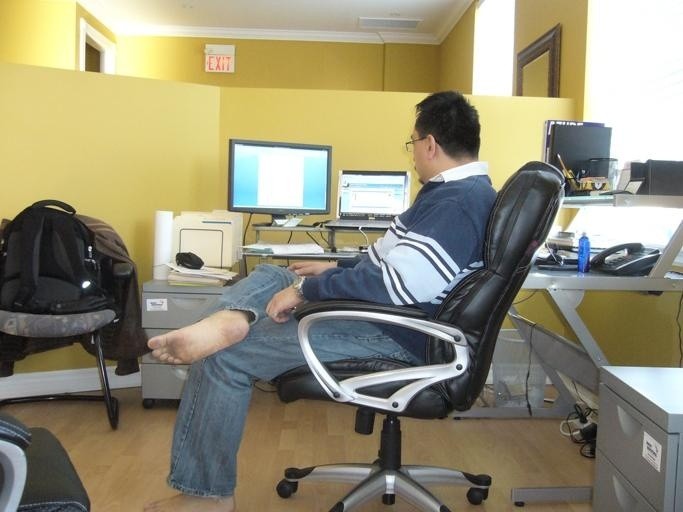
[0,409,90,512]
[277,160,565,512]
[0,212,132,431]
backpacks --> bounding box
[0,200,110,315]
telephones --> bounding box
[590,243,662,276]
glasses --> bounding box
[406,136,427,152]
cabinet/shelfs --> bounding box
[140,280,230,408]
[590,366,683,512]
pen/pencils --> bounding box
[556,154,579,191]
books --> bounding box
[543,120,606,164]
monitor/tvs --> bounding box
[228,138,332,227]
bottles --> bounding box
[578,232,591,273]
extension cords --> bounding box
[574,416,598,443]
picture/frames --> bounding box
[517,21,561,98]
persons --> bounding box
[142,92,495,512]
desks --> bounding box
[243,252,682,417]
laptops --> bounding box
[325,169,411,228]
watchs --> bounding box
[293,274,312,302]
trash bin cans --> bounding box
[492,329,547,408]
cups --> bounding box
[589,157,619,195]
